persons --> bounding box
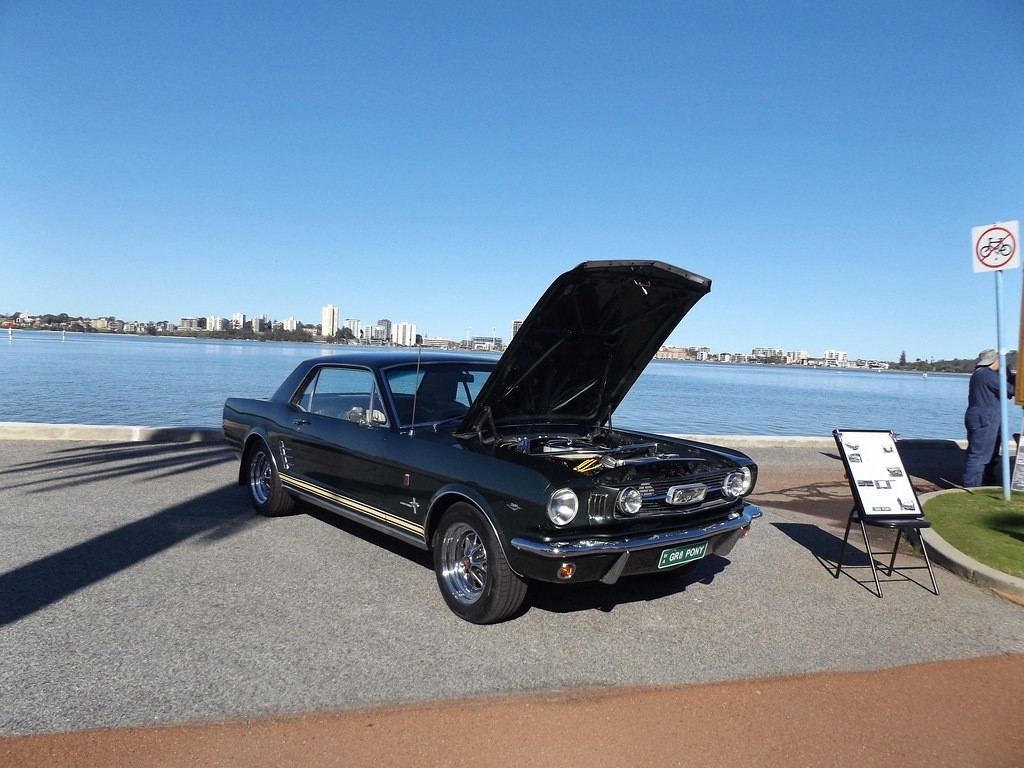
[962,350,1017,489]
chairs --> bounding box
[835,505,941,598]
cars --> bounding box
[224,259,764,626]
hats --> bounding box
[976,349,998,365]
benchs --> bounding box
[329,393,449,428]
